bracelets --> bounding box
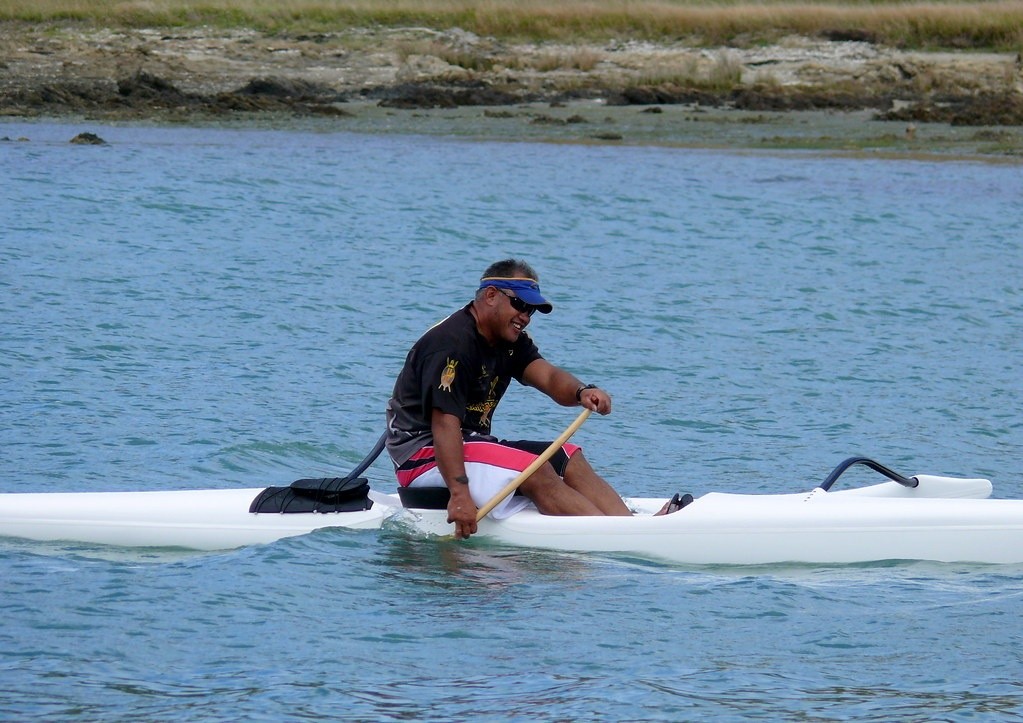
[576,383,599,405]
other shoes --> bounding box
[655,493,693,517]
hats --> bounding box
[478,275,555,316]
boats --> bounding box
[2,453,1021,578]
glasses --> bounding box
[492,284,539,319]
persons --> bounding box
[385,258,693,539]
